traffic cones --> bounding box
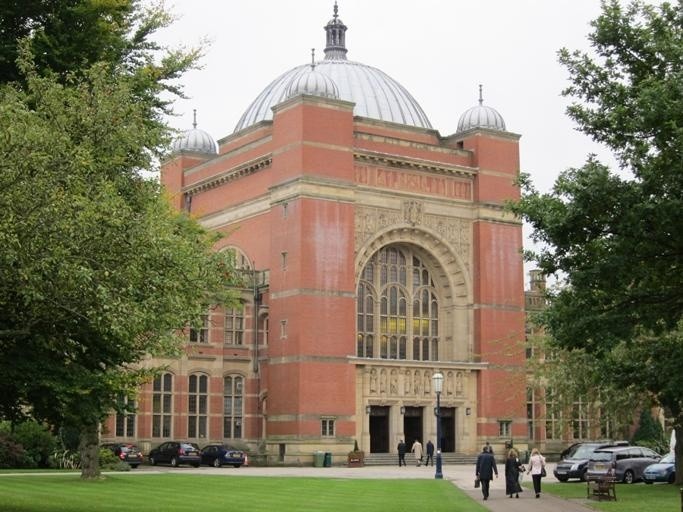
[243,453,249,467]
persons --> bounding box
[397,439,406,466]
[504,448,523,498]
[525,447,546,498]
[425,440,434,466]
[411,438,423,466]
[475,445,498,501]
[485,441,495,454]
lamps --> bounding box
[434,407,438,415]
[366,405,371,413]
[401,406,405,414]
[466,408,471,415]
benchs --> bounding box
[348,439,364,467]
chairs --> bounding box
[587,475,616,501]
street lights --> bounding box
[431,372,445,479]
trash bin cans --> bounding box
[313,452,331,467]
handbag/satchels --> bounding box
[541,466,546,477]
[474,476,480,488]
[517,464,526,472]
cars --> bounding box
[99,442,145,468]
[552,441,676,485]
[148,440,202,468]
[199,442,245,468]
